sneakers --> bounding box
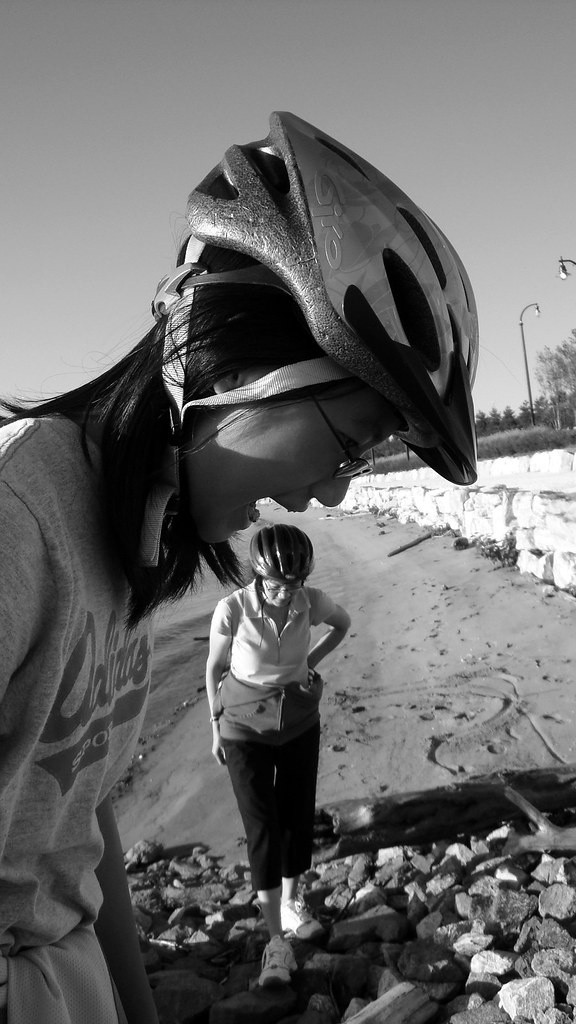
[280,898,324,939]
[259,936,298,987]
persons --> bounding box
[205,523,350,987]
[1,114,478,1024]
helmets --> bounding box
[250,525,313,582]
[185,112,480,486]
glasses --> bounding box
[310,397,370,482]
[260,581,302,594]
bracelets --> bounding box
[210,718,218,722]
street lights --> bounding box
[519,301,541,427]
